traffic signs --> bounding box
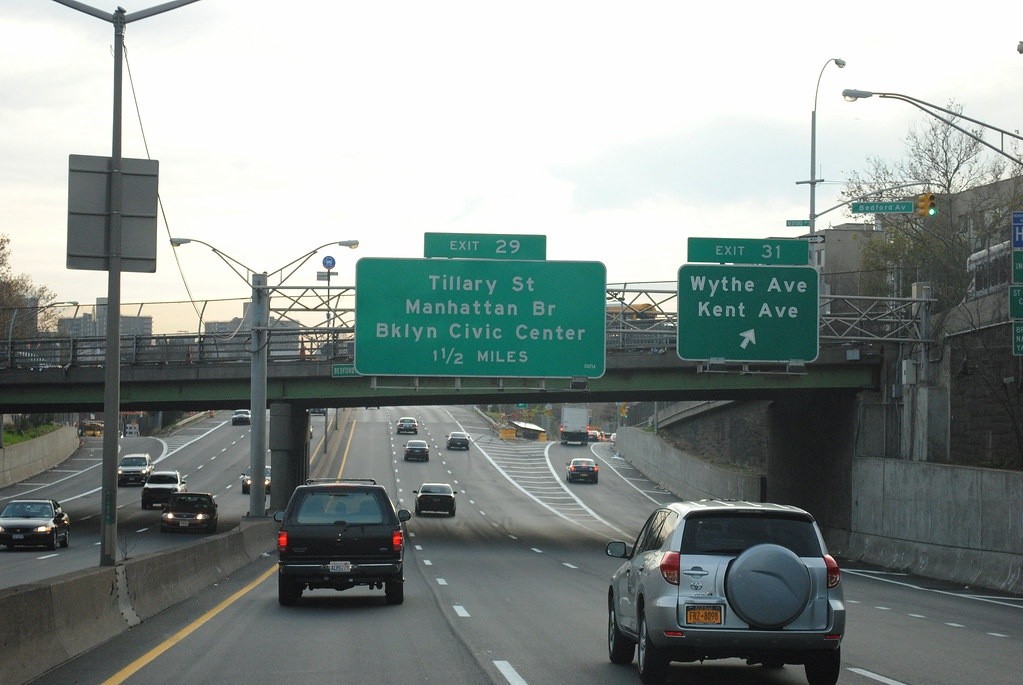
[677,265,820,364]
[799,235,826,243]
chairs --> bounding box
[303,498,346,513]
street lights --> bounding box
[810,59,845,234]
[169,237,359,518]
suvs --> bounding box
[118,454,185,508]
[605,499,845,685]
[274,478,411,604]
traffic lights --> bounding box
[926,192,935,215]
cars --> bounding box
[610,433,616,441]
[412,483,457,517]
[396,417,419,434]
[232,410,251,425]
[565,458,599,482]
[446,432,470,450]
[0,499,71,550]
[241,466,271,493]
[588,431,601,442]
[160,492,218,534]
[403,440,430,462]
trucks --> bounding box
[560,407,589,446]
